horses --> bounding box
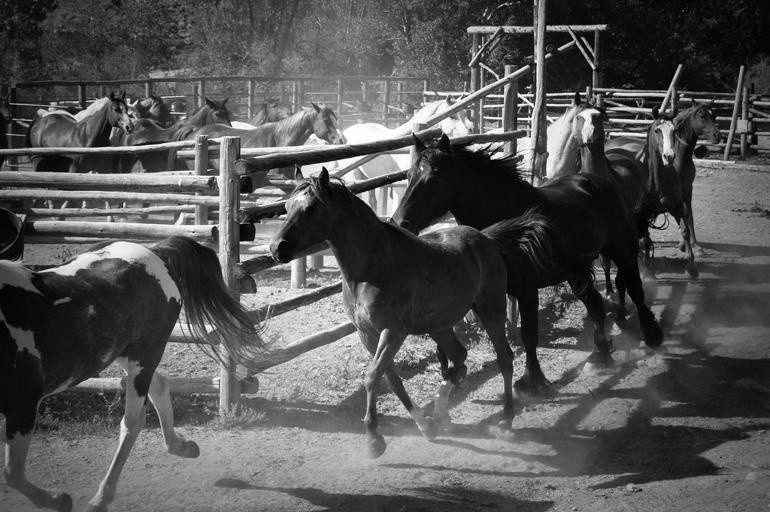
[268,164,554,461]
[0,237,273,511]
[385,132,667,397]
[0,90,722,281]
